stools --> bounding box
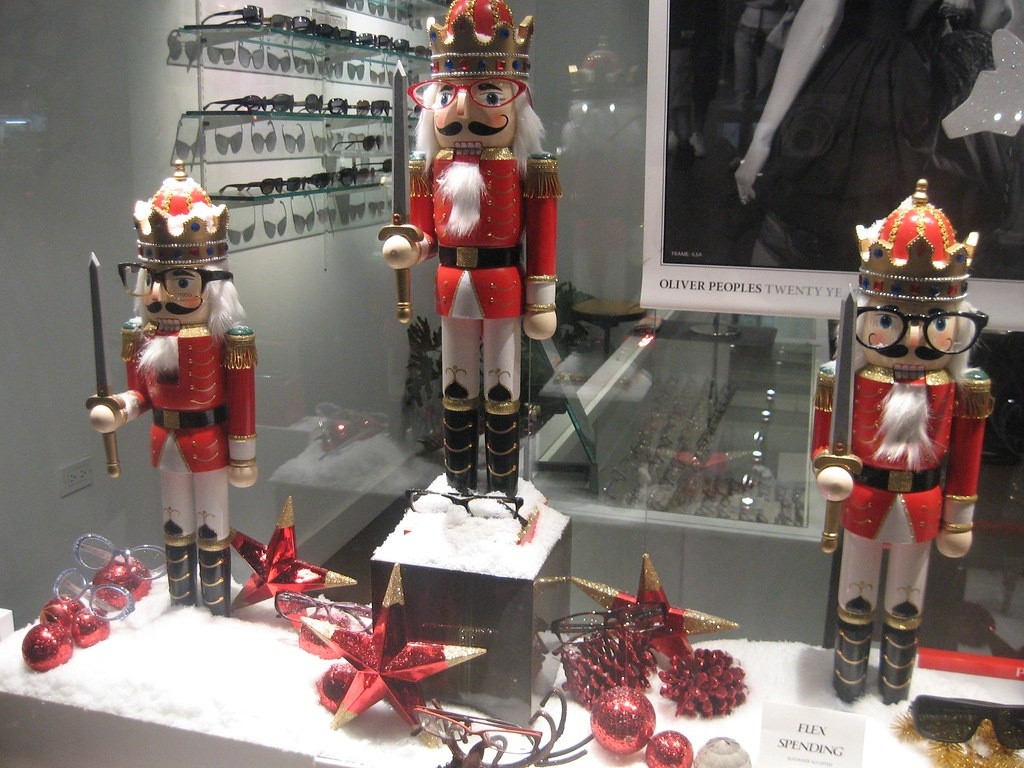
[570,296,646,495]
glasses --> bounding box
[413,705,542,755]
[325,0,413,22]
[203,94,390,117]
[118,261,235,298]
[225,195,393,245]
[854,306,989,355]
[406,489,528,527]
[168,29,419,90]
[332,135,383,151]
[53,568,136,620]
[550,601,672,656]
[72,534,168,581]
[407,77,533,110]
[601,374,805,527]
[912,695,1024,750]
[219,158,391,194]
[202,6,430,58]
[274,590,373,634]
[176,114,394,161]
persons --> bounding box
[378,0,564,498]
[810,178,994,705]
[89,160,258,617]
[668,0,1024,280]
